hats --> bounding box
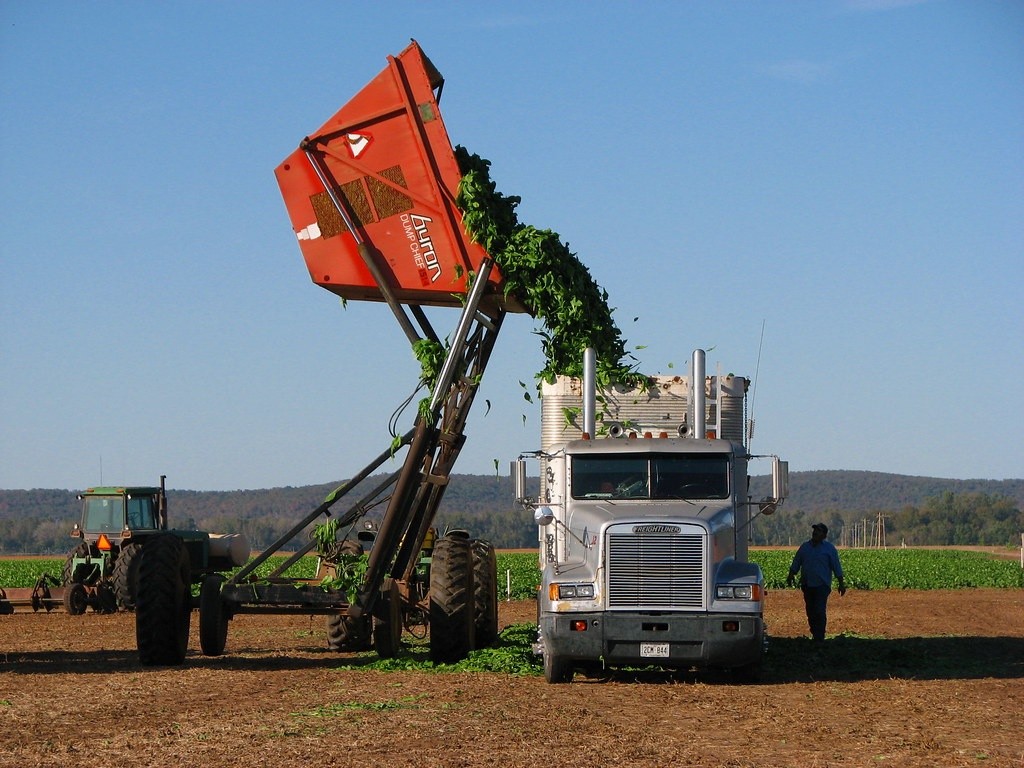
[812,523,828,534]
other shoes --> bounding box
[815,640,824,647]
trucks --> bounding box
[507,343,792,685]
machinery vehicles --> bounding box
[59,474,251,616]
[133,34,536,668]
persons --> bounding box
[787,523,846,661]
